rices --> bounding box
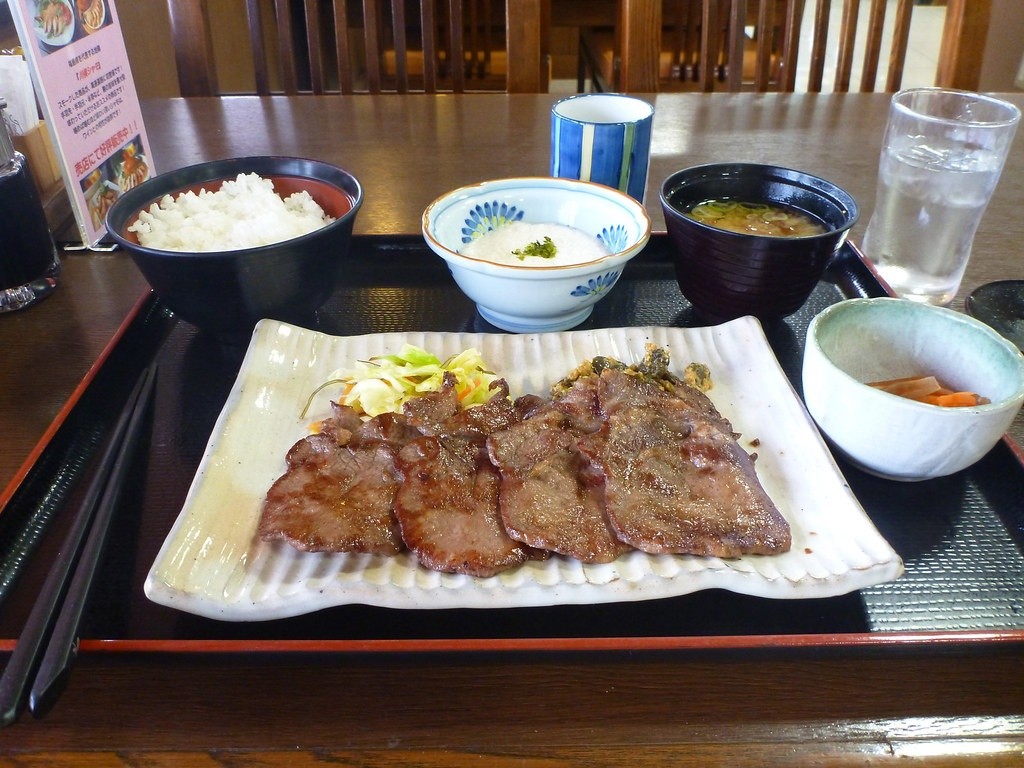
[127,171,338,252]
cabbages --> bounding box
[328,345,504,420]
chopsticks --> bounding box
[0,361,160,731]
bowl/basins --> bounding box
[106,155,364,340]
[421,179,652,334]
[660,163,1024,482]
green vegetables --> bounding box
[511,235,558,262]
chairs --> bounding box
[166,0,541,96]
[622,0,992,97]
[576,27,784,96]
[376,41,553,96]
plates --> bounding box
[965,279,1024,355]
[32,0,105,46]
[144,317,908,620]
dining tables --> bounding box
[0,96,1024,768]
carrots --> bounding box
[883,376,977,408]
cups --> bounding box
[860,86,1021,306]
[549,93,654,207]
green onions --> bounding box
[683,199,790,235]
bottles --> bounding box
[0,98,61,314]
